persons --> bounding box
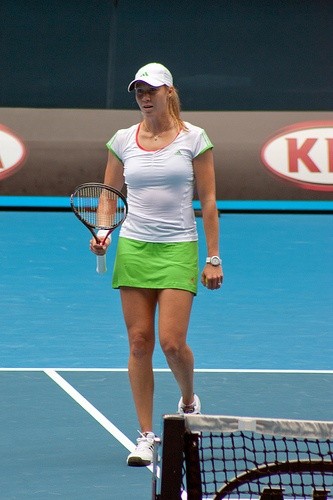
[90,63,223,467]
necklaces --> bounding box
[143,117,171,141]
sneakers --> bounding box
[178,394,200,447]
[127,430,160,466]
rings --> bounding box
[218,283,222,285]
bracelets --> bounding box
[97,230,111,242]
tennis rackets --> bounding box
[70,181,129,275]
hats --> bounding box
[128,63,173,93]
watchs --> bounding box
[206,256,223,266]
